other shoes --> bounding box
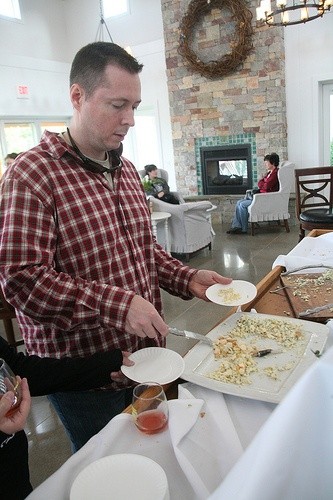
[226,228,238,234]
[236,231,247,235]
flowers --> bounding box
[141,174,158,192]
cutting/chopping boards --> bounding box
[178,312,329,403]
[281,272,333,319]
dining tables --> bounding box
[15,228,333,500]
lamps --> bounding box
[255,0,333,27]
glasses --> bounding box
[66,127,123,174]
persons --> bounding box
[226,153,280,233]
[0,349,135,500]
[4,152,22,168]
[0,43,232,455]
[143,165,179,204]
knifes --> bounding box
[298,304,333,316]
[169,328,212,344]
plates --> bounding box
[205,279,258,308]
[121,346,185,387]
[69,454,171,500]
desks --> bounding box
[149,211,173,258]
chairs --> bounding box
[295,167,333,242]
[247,162,293,236]
[136,167,185,204]
[147,193,215,263]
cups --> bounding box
[132,381,168,434]
[0,359,22,416]
